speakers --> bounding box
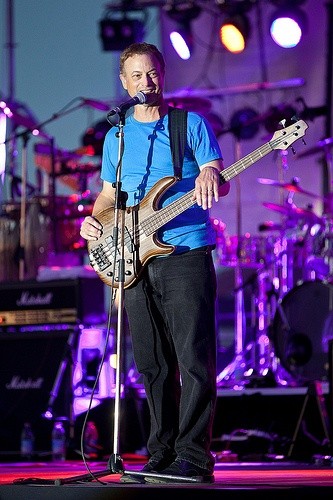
[0,325,81,459]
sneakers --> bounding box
[120,452,171,483]
[144,459,215,483]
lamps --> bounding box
[219,0,252,56]
[167,5,202,62]
[268,0,309,50]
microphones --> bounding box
[294,138,333,159]
[82,97,110,111]
[107,92,148,116]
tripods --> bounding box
[55,126,214,484]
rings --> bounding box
[208,190,213,191]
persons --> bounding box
[79,44,231,484]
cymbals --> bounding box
[256,176,328,201]
[262,201,329,227]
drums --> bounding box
[30,196,94,266]
[0,202,41,284]
[270,282,333,386]
[213,216,333,297]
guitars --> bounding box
[86,115,309,290]
